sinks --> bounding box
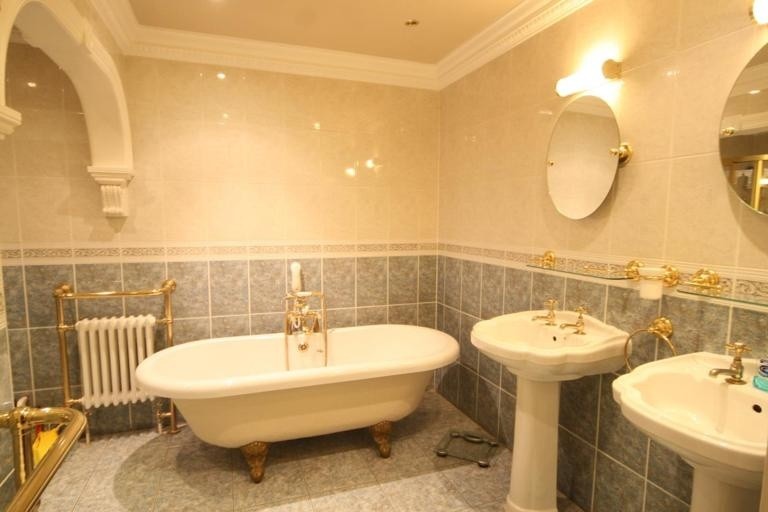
[612,352,767,488]
[472,309,631,380]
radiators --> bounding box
[75,313,158,410]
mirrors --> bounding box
[717,42,768,217]
[544,96,632,221]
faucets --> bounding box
[532,299,559,324]
[560,307,586,334]
[710,341,753,383]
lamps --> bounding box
[747,2,767,23]
[552,57,623,98]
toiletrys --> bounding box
[755,359,768,389]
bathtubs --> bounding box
[135,324,460,450]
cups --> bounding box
[638,267,665,301]
